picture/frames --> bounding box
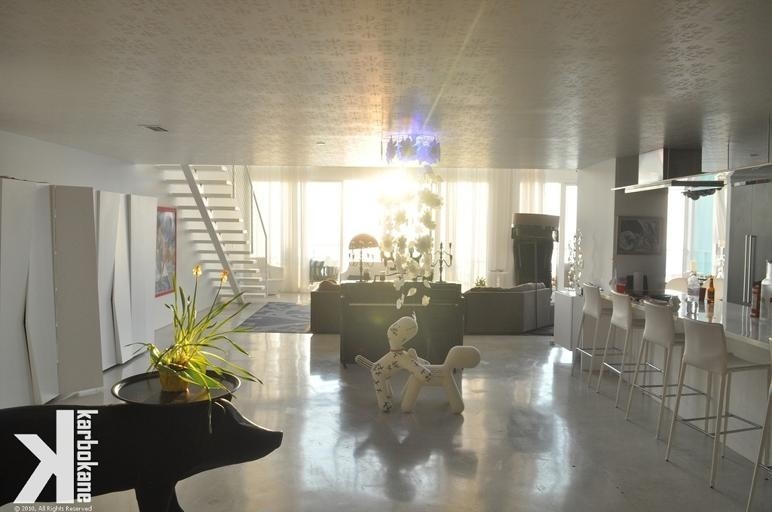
[615,215,664,257]
[154,206,177,298]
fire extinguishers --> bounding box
[749,282,760,318]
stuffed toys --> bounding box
[355,316,432,413]
[400,345,481,415]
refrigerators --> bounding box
[726,183,772,305]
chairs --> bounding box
[570,281,771,511]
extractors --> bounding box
[612,149,725,202]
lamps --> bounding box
[380,132,444,310]
[347,233,379,283]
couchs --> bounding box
[311,280,551,339]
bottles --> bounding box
[686,273,715,304]
[750,260,772,323]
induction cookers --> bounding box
[646,292,679,306]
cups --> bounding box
[364,269,370,282]
[379,271,386,280]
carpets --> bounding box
[232,302,311,334]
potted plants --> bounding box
[117,263,265,436]
